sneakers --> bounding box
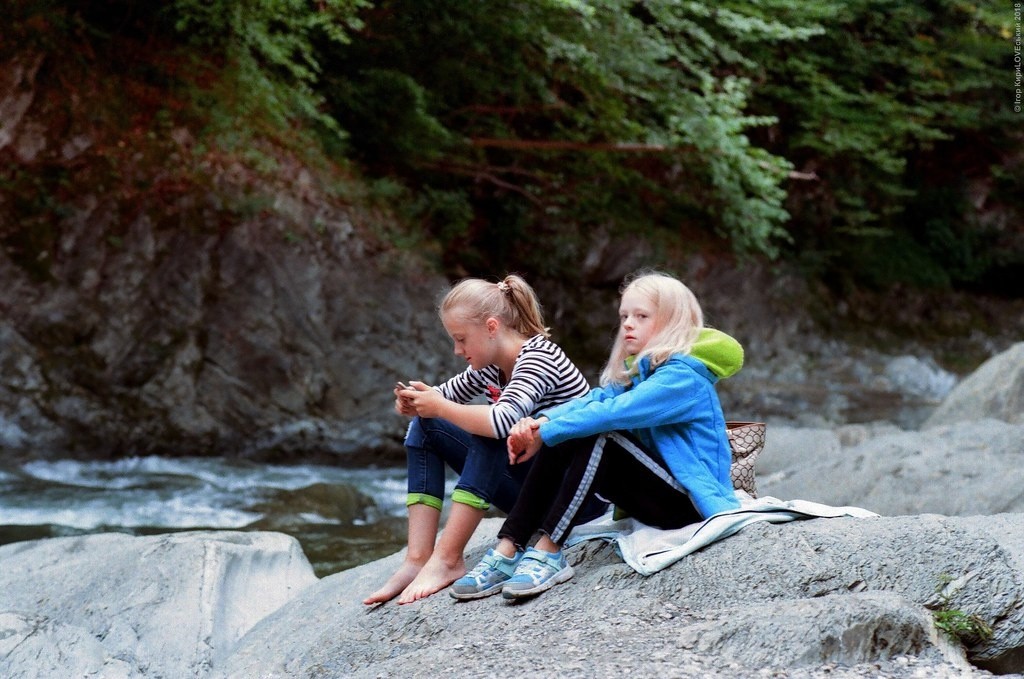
[503,545,577,599]
[448,546,522,599]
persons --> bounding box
[363,273,591,605]
[447,273,744,599]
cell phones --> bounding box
[396,382,406,391]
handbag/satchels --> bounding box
[724,419,768,501]
